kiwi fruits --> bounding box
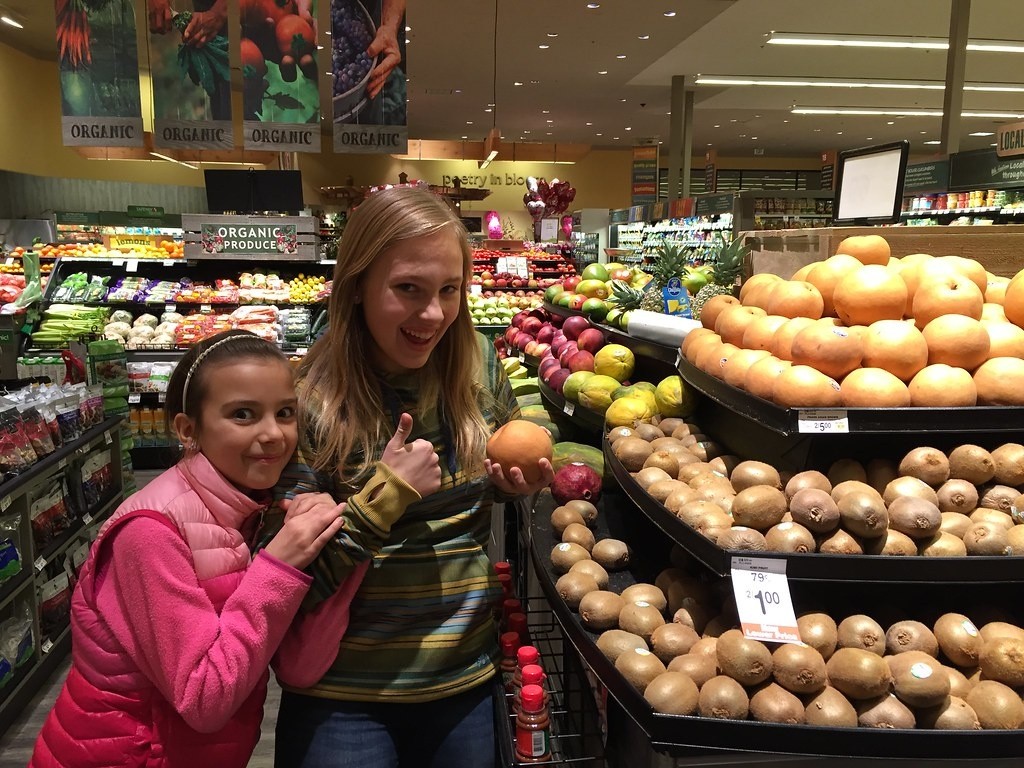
[549,500,1024,730]
[604,418,1024,556]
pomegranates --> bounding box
[551,462,603,506]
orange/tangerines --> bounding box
[544,261,713,326]
[0,240,184,273]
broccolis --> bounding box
[97,310,184,350]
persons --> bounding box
[20,328,370,768]
[248,181,555,768]
[366,0,405,98]
[146,0,233,121]
[257,0,319,83]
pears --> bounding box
[486,420,553,483]
[290,272,326,304]
[682,234,1024,409]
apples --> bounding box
[463,271,547,325]
[493,307,631,398]
[473,249,575,273]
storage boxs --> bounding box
[109,235,174,249]
[483,240,524,252]
[181,213,321,261]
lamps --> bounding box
[391,0,593,169]
[70,0,278,170]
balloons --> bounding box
[484,210,503,240]
[522,176,576,239]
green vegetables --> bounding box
[30,303,111,349]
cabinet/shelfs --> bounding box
[570,233,599,274]
[505,300,1024,768]
[0,413,128,741]
[740,191,832,232]
[468,260,576,337]
[617,223,643,268]
[642,214,733,273]
[23,257,339,469]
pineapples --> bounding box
[610,231,752,322]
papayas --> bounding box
[506,344,694,481]
